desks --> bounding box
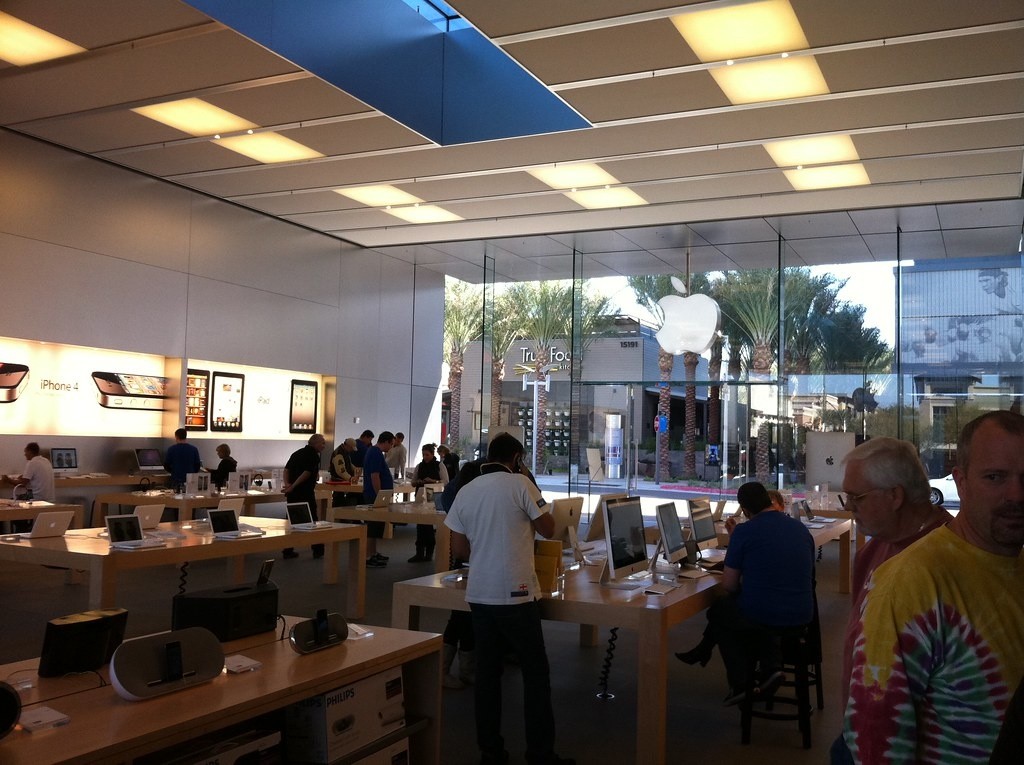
[0,463,865,765]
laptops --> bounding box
[402,487,424,505]
[207,509,263,538]
[838,494,845,506]
[433,492,446,513]
[722,506,742,517]
[356,490,393,508]
[202,498,244,523]
[104,515,167,549]
[800,500,836,523]
[17,511,74,538]
[793,502,825,529]
[713,501,727,520]
[285,502,331,530]
[133,504,165,529]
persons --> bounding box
[441,432,505,689]
[281,434,326,559]
[830,437,953,765]
[2,442,55,533]
[842,409,1024,765]
[408,445,449,563]
[443,433,575,765]
[362,431,396,567]
[205,444,237,491]
[903,268,1024,361]
[676,482,816,707]
[437,445,460,480]
[165,429,200,521]
[351,430,374,468]
[329,439,358,506]
[384,433,406,479]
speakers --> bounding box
[38,605,128,678]
[171,579,279,642]
[289,613,349,655]
[109,626,225,701]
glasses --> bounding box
[843,488,882,505]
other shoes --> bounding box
[527,755,575,765]
[313,550,324,558]
[283,548,299,558]
[480,750,510,765]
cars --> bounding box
[927,474,962,505]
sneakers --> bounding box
[759,661,785,700]
[374,552,389,561]
[366,556,387,567]
[723,669,759,706]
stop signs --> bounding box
[653,416,660,432]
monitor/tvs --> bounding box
[50,447,79,473]
[686,497,718,552]
[583,493,627,542]
[134,448,165,470]
[805,431,856,498]
[599,496,649,590]
[551,497,583,562]
[649,502,688,574]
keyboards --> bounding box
[708,561,724,571]
[678,570,710,579]
[644,583,674,595]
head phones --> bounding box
[254,474,263,486]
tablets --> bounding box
[185,368,210,431]
[210,371,245,432]
[289,379,318,434]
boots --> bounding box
[408,544,424,562]
[449,649,478,685]
[424,545,434,561]
[442,643,465,688]
[675,636,716,667]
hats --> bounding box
[345,439,357,451]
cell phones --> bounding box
[257,559,275,585]
[198,475,208,490]
[165,640,183,682]
[239,474,248,488]
[317,609,330,644]
[516,456,522,474]
[91,372,165,411]
[268,481,272,489]
[0,362,30,403]
[28,488,34,501]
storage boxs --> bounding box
[289,667,408,765]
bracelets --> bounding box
[917,505,934,532]
